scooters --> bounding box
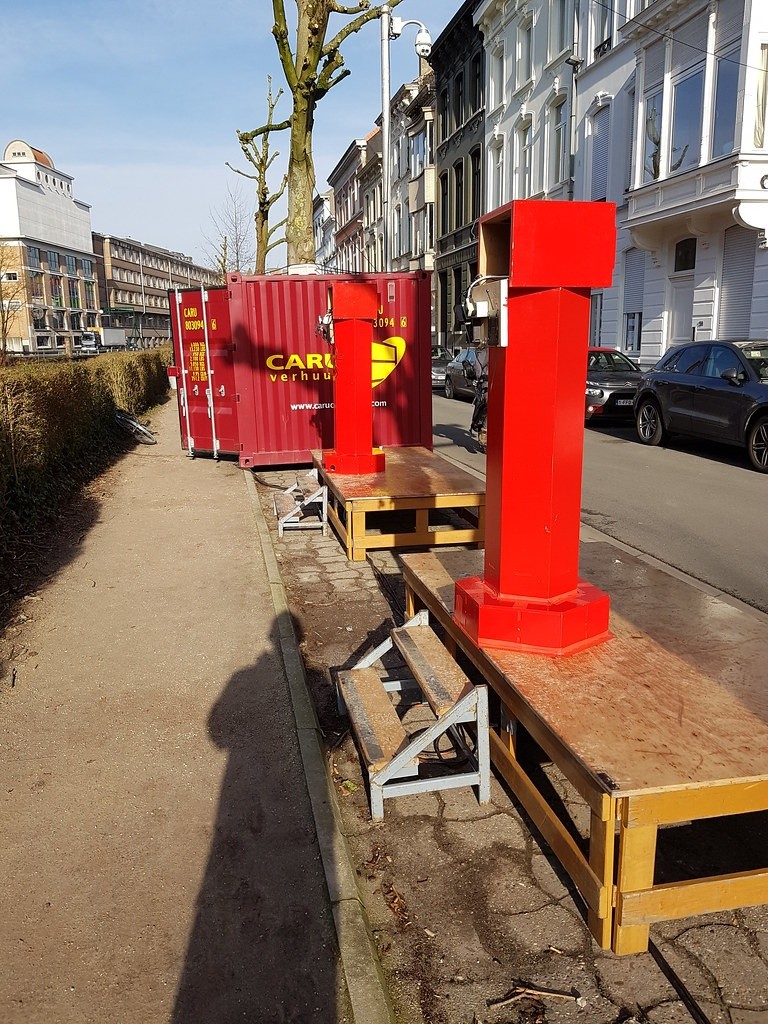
[470,375,488,452]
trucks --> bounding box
[81,326,126,352]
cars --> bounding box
[445,347,489,400]
[431,344,456,388]
[632,338,767,472]
[585,347,644,423]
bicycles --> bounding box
[114,407,157,444]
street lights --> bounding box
[378,5,433,270]
